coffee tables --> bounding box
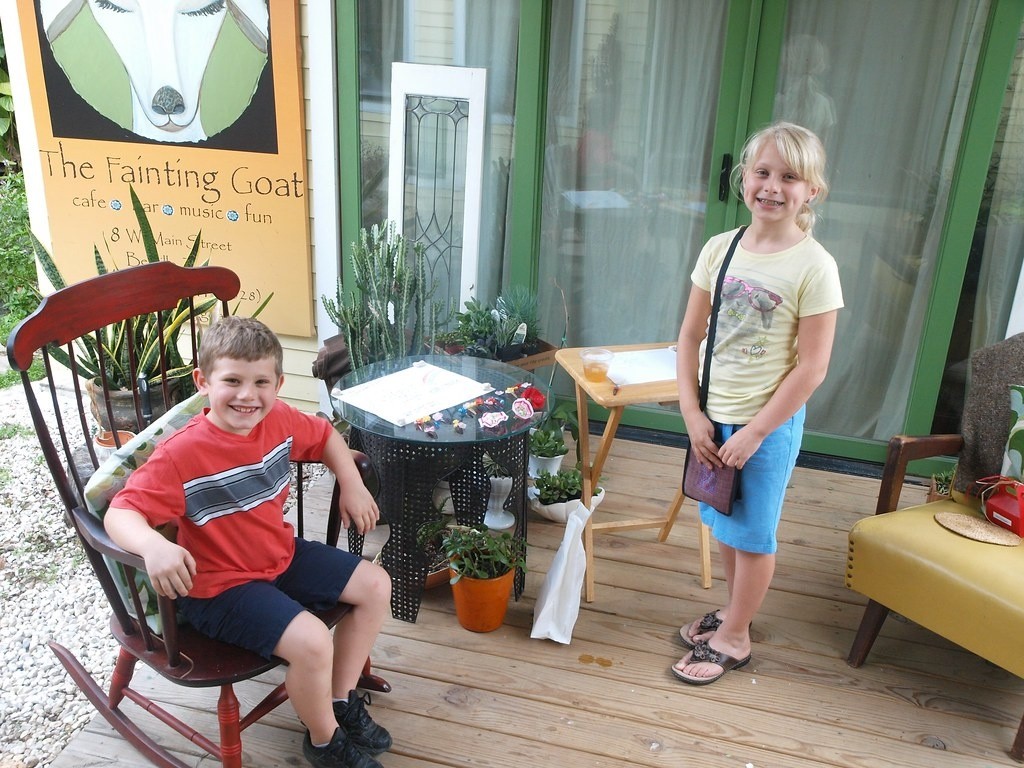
[329,354,555,623]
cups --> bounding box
[579,348,613,382]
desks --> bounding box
[554,341,711,604]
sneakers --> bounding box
[302,727,383,768]
[333,689,392,754]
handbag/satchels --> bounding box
[681,440,742,516]
[529,500,594,645]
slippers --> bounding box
[679,609,753,648]
[670,639,752,685]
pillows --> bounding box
[85,388,191,635]
[1000,382,1024,496]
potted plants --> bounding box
[373,497,484,588]
[527,464,605,523]
[20,183,274,432]
[927,468,955,502]
[527,397,580,480]
[426,287,541,360]
[482,459,515,530]
[424,464,469,514]
[439,524,528,634]
[528,274,570,442]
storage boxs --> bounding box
[986,479,1024,539]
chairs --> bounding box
[6,263,392,768]
[844,332,1024,762]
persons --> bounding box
[103,314,393,767]
[672,123,843,683]
